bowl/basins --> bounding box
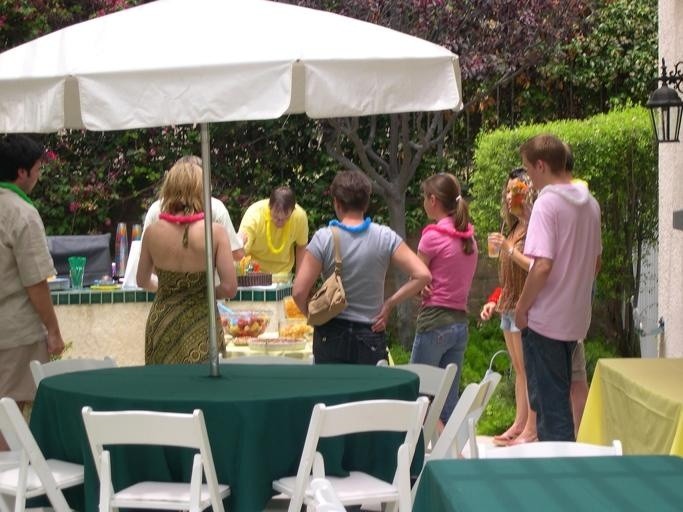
[219,307,273,345]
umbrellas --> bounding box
[0,0,464,380]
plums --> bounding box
[221,316,264,337]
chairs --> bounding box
[265,395,430,512]
[399,367,502,507]
[468,434,625,459]
[215,351,317,370]
[76,400,232,512]
[0,394,87,512]
[373,356,460,456]
[26,353,117,390]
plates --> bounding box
[248,337,305,351]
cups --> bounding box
[114,222,142,278]
[66,256,87,289]
[487,233,499,257]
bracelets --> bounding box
[508,247,514,256]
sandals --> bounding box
[492,429,538,447]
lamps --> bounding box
[635,53,682,146]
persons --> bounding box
[291,170,433,366]
[238,186,310,281]
[478,168,539,448]
[560,140,590,439]
[104,182,140,259]
[135,162,238,368]
[511,134,604,441]
[1,133,66,457]
[410,173,478,460]
[140,154,248,287]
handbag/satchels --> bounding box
[306,272,349,326]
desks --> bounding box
[26,363,425,512]
[413,449,681,512]
